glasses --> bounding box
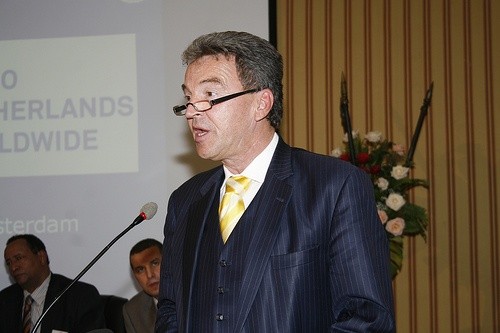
[172,87,263,117]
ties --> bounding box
[219,175,251,246]
[22,295,35,333]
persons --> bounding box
[123,239,163,333]
[0,232,107,333]
[155,31,398,333]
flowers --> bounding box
[326,128,433,281]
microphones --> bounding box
[31,201,158,333]
